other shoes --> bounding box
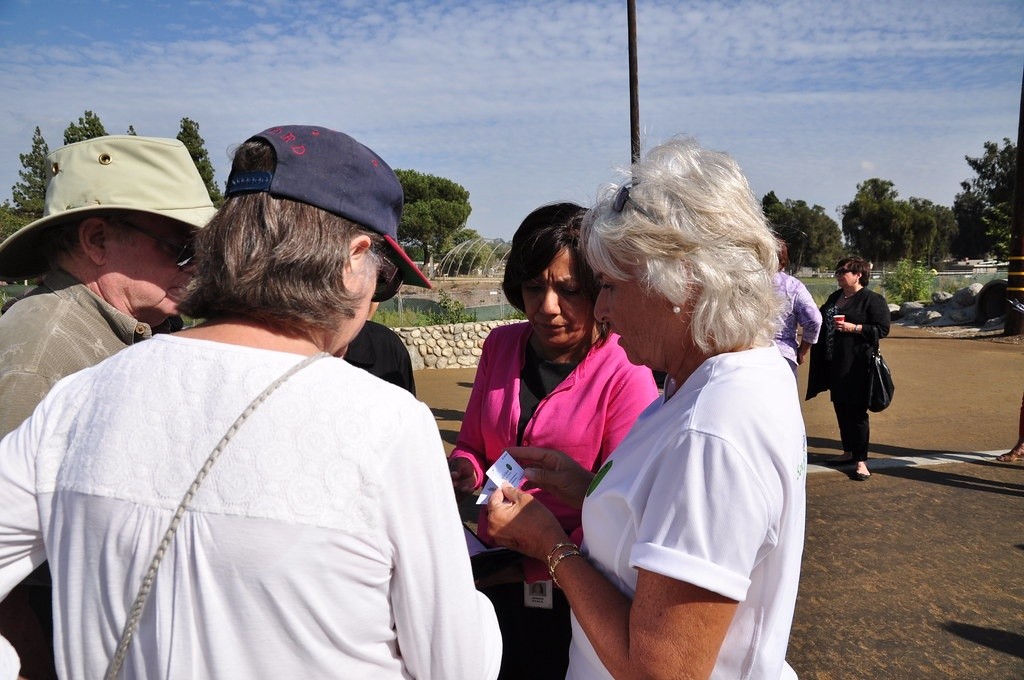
[824,454,854,466]
[854,460,871,481]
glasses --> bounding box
[102,216,213,286]
[834,268,859,276]
[371,246,407,302]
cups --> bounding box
[833,315,845,322]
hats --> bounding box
[223,125,432,290]
[0,135,220,281]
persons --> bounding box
[532,583,543,595]
[805,256,891,479]
[772,239,822,383]
[479,133,808,680]
[996,393,1024,463]
[444,203,661,680]
[0,124,505,679]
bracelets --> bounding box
[547,543,581,589]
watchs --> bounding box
[855,324,858,333]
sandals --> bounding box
[997,444,1024,461]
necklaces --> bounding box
[843,293,853,298]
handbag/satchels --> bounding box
[864,325,894,412]
